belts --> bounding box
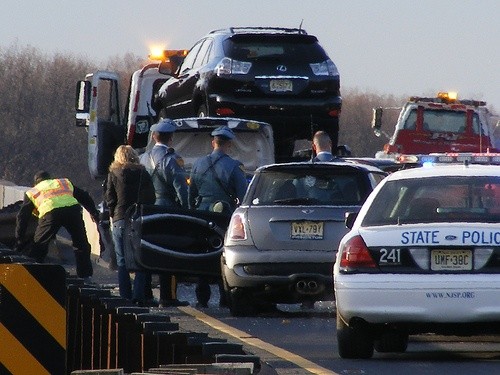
[156,193,175,199]
[202,196,231,201]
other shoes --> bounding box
[160,300,188,306]
[196,285,211,303]
[138,301,158,306]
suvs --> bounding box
[152,17,343,163]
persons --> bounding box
[16,170,102,278]
[188,126,247,308]
[106,145,160,307]
[293,131,352,203]
[137,122,190,307]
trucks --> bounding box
[369,91,500,161]
[73,25,353,188]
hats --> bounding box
[211,126,234,139]
[150,123,174,132]
[159,119,176,125]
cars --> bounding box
[332,162,500,361]
[121,160,390,312]
